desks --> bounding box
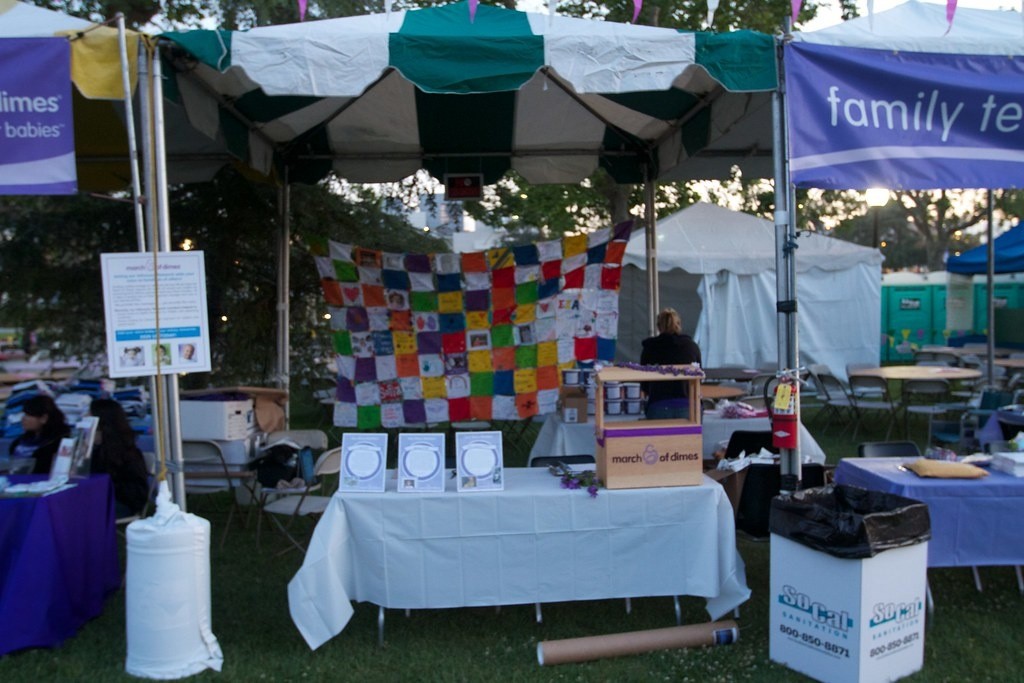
[333,462,723,646]
[0,472,112,655]
[841,455,1024,614]
[549,409,799,462]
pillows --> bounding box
[901,459,989,477]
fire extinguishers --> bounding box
[763,366,807,449]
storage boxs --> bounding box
[770,484,930,683]
[594,365,705,489]
[559,386,589,423]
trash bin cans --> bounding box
[769,481,931,683]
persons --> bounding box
[7,393,72,472]
[641,305,703,419]
[88,396,147,517]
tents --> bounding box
[0,2,165,486]
[946,221,1024,274]
[665,2,1024,494]
[621,200,885,391]
[142,0,793,511]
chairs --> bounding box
[242,429,328,554]
[182,438,254,556]
[262,446,344,566]
[701,339,1024,441]
[116,452,156,595]
[858,440,983,594]
